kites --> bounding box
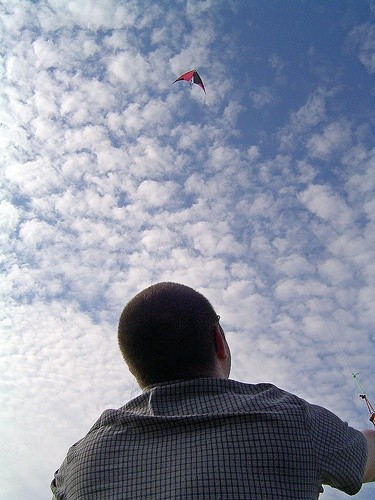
[172,69,208,95]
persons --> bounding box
[49,281,375,500]
